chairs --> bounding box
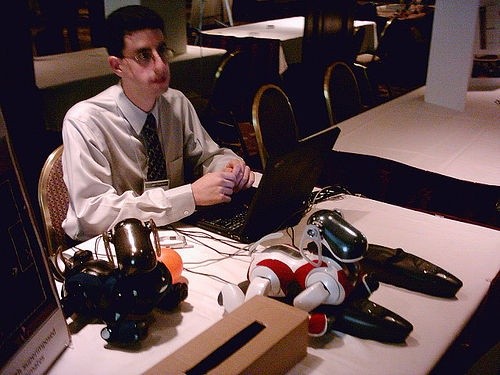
[37,0,361,266]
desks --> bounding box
[298,77,500,231]
[34,45,228,133]
[198,16,380,83]
[37,170,500,375]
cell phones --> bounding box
[159,236,194,249]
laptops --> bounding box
[181,125,341,243]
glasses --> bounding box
[125,47,177,66]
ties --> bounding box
[137,114,169,192]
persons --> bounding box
[62,5,255,242]
